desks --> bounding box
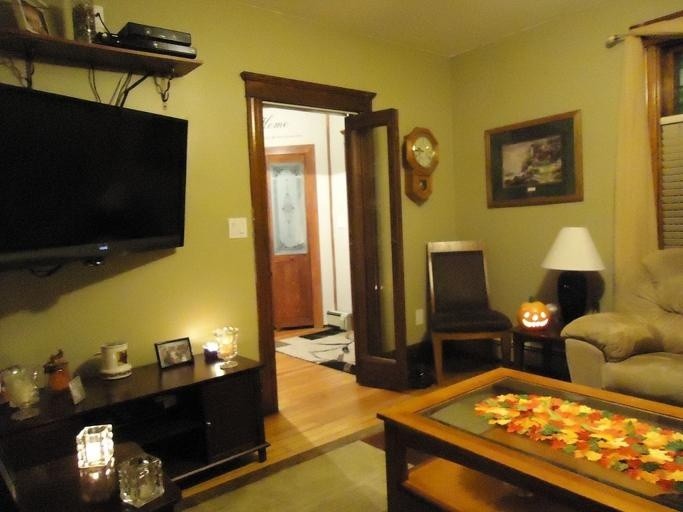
[0,351,271,512]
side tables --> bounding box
[510,324,563,380]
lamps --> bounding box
[541,226,605,325]
[516,296,551,331]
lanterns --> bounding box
[516,294,554,332]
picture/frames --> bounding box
[483,110,582,209]
[154,337,195,369]
[12,0,57,39]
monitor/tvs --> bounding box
[0,82,189,264]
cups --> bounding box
[76,424,114,469]
[98,340,132,381]
[117,453,164,506]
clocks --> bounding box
[405,127,440,201]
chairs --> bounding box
[559,246,682,409]
[427,239,513,387]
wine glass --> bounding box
[4,368,41,421]
[212,326,240,369]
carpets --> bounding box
[173,388,501,511]
[275,327,357,376]
